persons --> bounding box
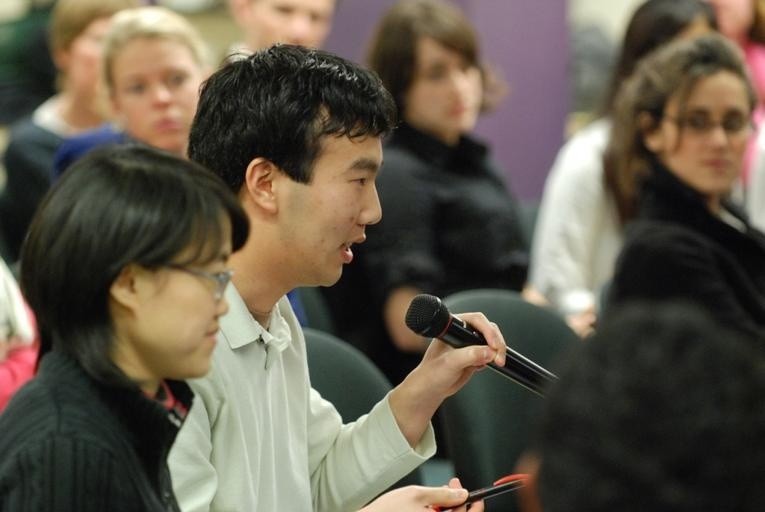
[602,28,764,326]
[0,1,156,260]
[48,6,210,178]
[226,1,337,51]
[518,299,764,511]
[520,0,718,328]
[0,1,59,127]
[0,143,252,512]
[166,41,506,511]
[709,0,764,233]
[361,1,533,355]
[0,255,42,410]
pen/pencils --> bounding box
[424,474,530,512]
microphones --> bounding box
[404,293,560,399]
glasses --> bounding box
[163,259,230,301]
